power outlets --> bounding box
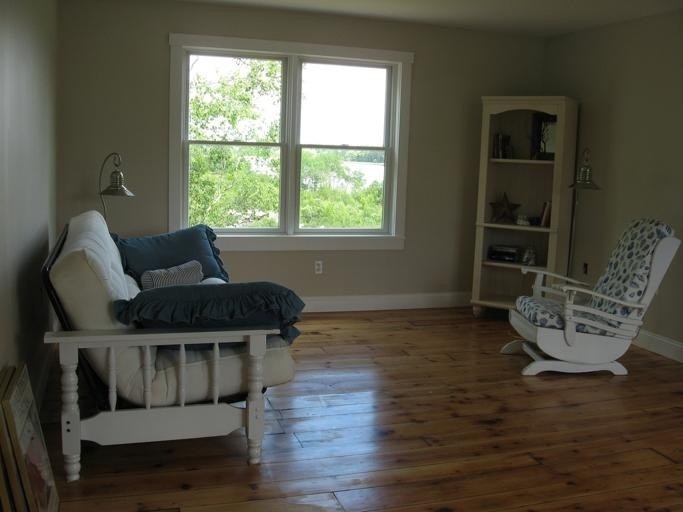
[314,260,323,274]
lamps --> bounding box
[566,147,602,277]
[98,152,136,222]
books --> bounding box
[538,200,550,228]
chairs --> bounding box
[499,218,682,377]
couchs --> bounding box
[41,208,297,484]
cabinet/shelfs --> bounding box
[470,95,581,319]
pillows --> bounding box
[110,224,305,345]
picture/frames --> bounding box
[532,112,557,161]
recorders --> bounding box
[487,246,522,262]
